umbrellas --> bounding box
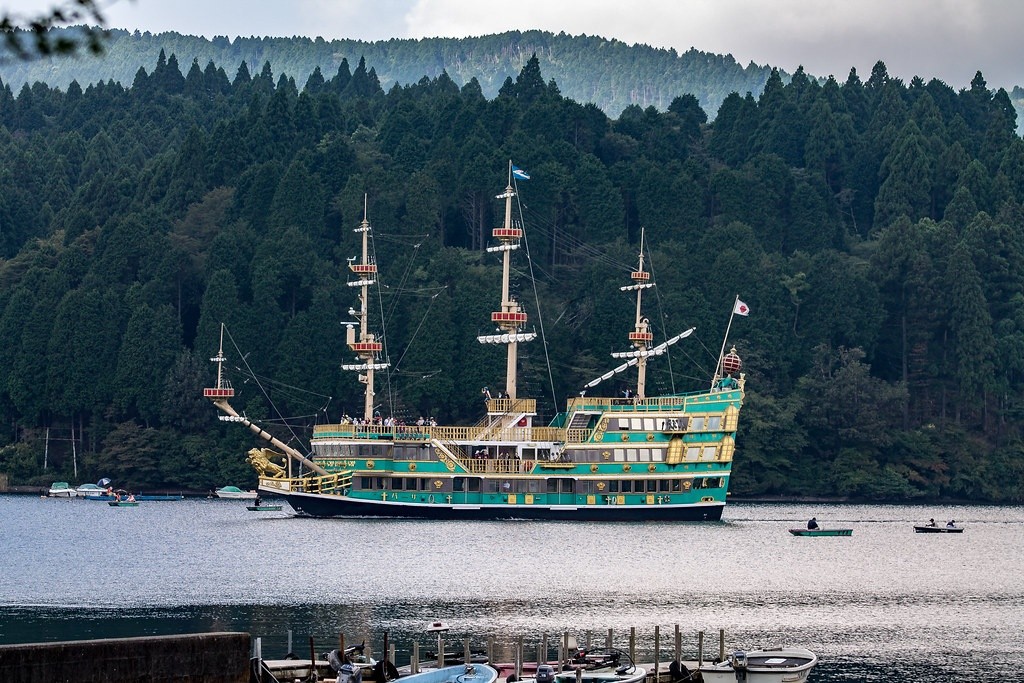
[97,478,111,487]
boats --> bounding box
[915,525,963,533]
[215,486,258,499]
[49,483,77,498]
[246,505,282,510]
[207,496,213,499]
[108,503,140,507]
[791,528,851,537]
[75,484,108,498]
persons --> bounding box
[504,481,511,492]
[340,414,439,433]
[481,387,511,411]
[947,520,957,528]
[107,487,135,502]
[926,519,937,527]
[808,517,820,530]
[474,448,573,473]
[622,389,634,404]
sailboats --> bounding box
[207,159,748,521]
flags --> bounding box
[734,299,750,316]
[512,164,530,180]
